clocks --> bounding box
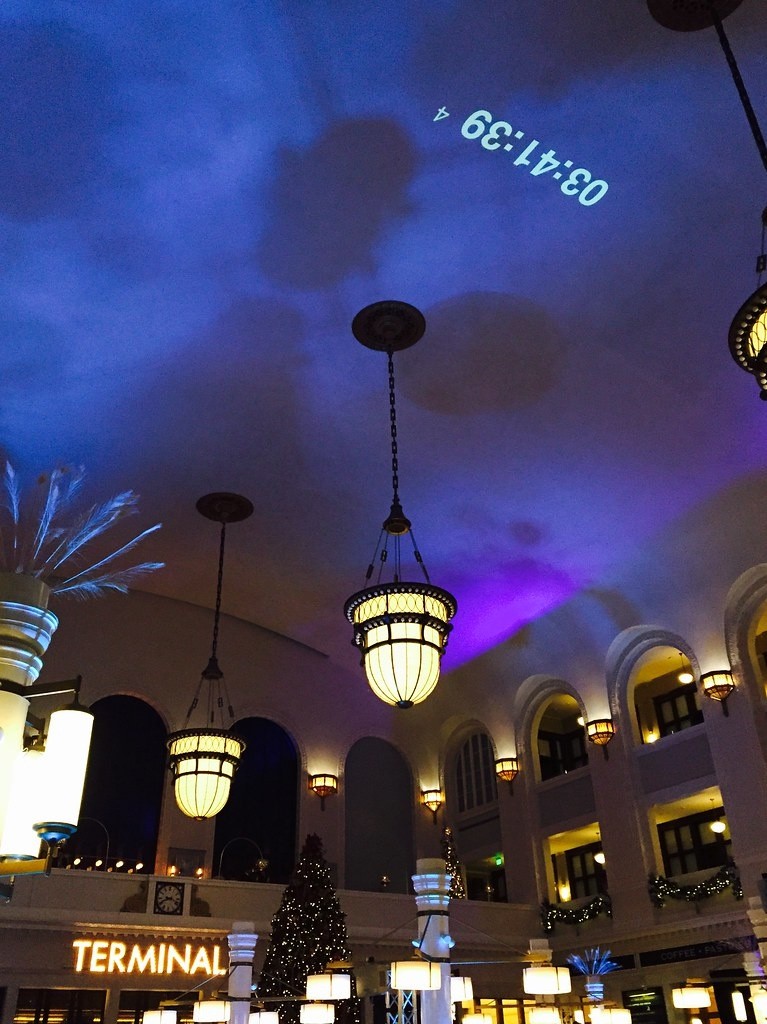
[154,881,184,915]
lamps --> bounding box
[193,963,238,1022]
[73,855,83,865]
[306,974,351,1000]
[342,302,457,711]
[308,773,339,812]
[586,718,615,761]
[731,983,750,1022]
[672,952,743,1009]
[643,0,767,402]
[679,653,693,684]
[421,790,442,825]
[142,967,223,1024]
[449,916,572,995]
[450,976,473,1003]
[381,875,391,893]
[484,884,496,903]
[252,965,335,1024]
[94,857,103,867]
[391,911,441,991]
[161,492,256,823]
[0,675,98,878]
[116,858,124,867]
[136,859,143,870]
[56,817,110,872]
[700,671,735,717]
[248,992,279,1024]
[495,758,518,796]
[212,837,269,881]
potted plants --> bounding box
[565,945,623,985]
[1,458,167,613]
[716,928,760,962]
